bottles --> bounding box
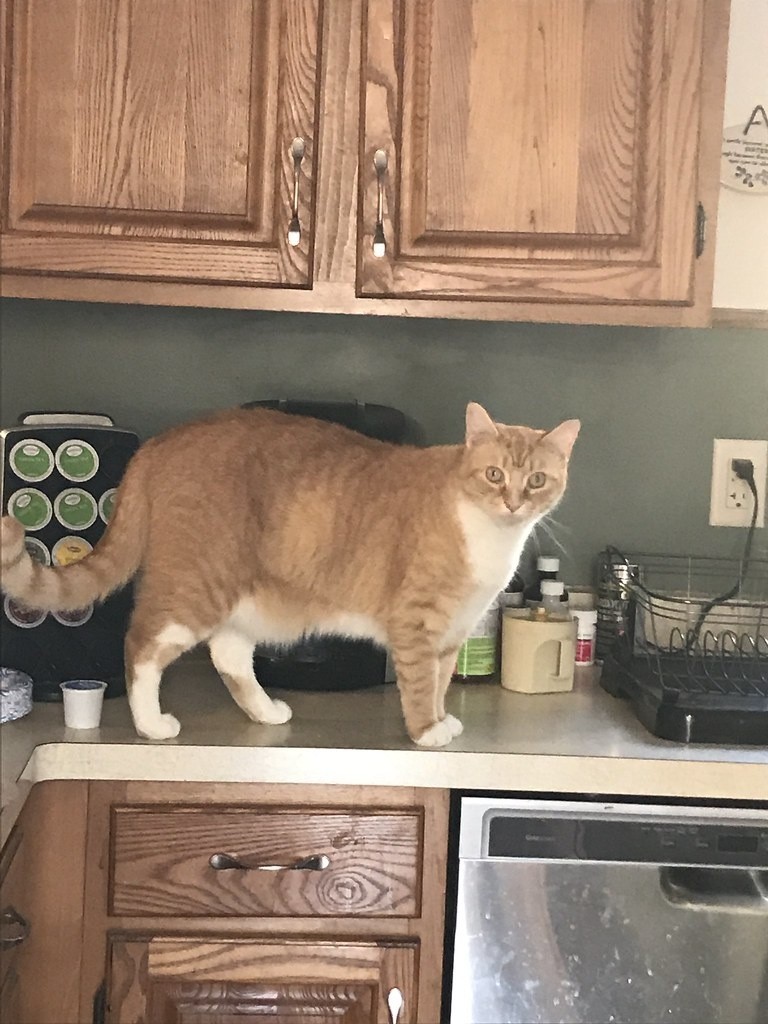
[453,556,598,683]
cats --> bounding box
[0,401,583,748]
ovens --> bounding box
[446,793,768,1024]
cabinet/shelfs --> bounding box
[0,1,734,328]
[0,779,452,1024]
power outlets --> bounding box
[708,438,768,530]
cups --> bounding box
[58,679,108,730]
